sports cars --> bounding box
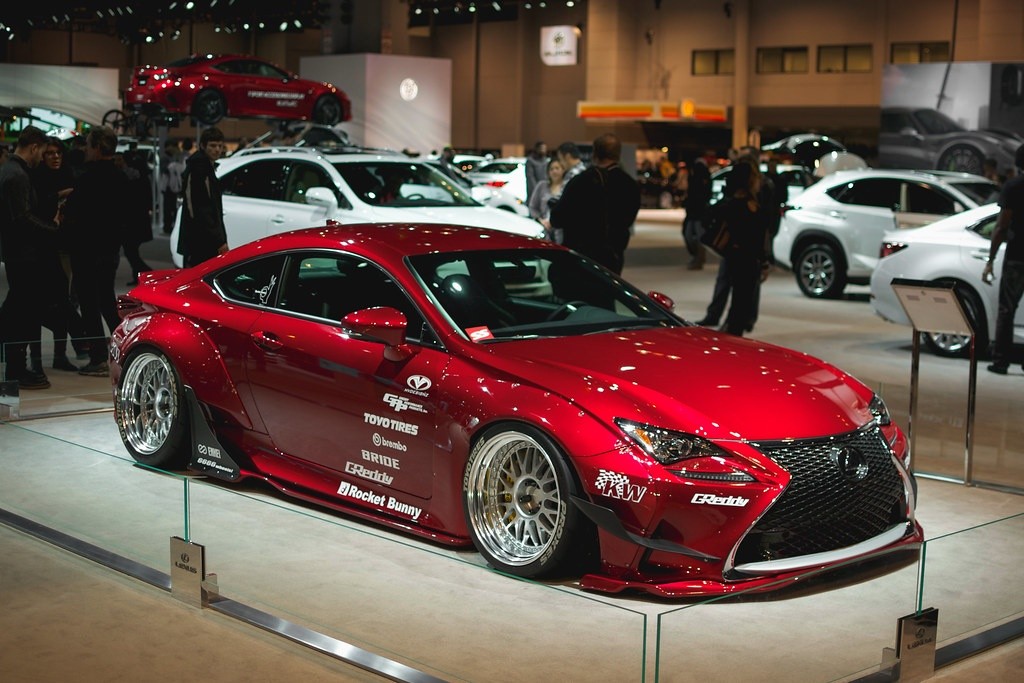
[105,220,926,601]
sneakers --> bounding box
[79,363,109,376]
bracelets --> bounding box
[988,257,995,261]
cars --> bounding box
[170,146,555,299]
[125,52,352,129]
[773,169,1000,299]
[398,154,551,219]
[708,135,869,218]
[875,106,1024,186]
[870,204,1024,358]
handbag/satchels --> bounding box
[700,219,729,258]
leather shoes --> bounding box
[53,357,77,371]
[6,366,31,385]
[30,361,42,373]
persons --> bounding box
[0,125,154,388]
[644,146,788,337]
[525,141,586,244]
[443,147,455,162]
[548,133,641,313]
[982,144,1024,375]
[161,137,193,233]
[177,126,229,267]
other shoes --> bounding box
[987,365,1008,374]
[689,258,704,270]
[127,277,139,287]
[694,320,708,325]
